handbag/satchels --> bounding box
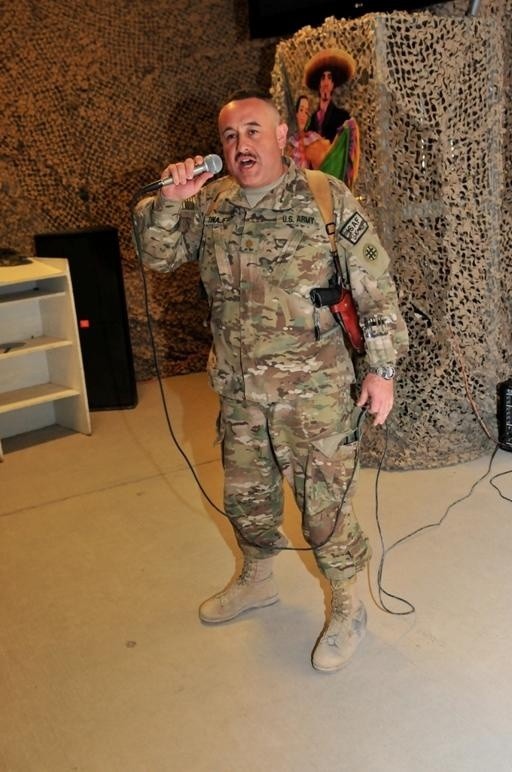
[329,287,365,355]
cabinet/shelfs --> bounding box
[0,257,97,456]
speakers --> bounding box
[33,226,138,410]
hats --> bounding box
[303,48,355,91]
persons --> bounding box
[281,47,362,187]
[130,88,411,670]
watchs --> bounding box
[368,366,396,380]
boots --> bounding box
[199,557,279,623]
[312,575,367,672]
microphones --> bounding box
[141,154,223,193]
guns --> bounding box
[310,286,339,307]
